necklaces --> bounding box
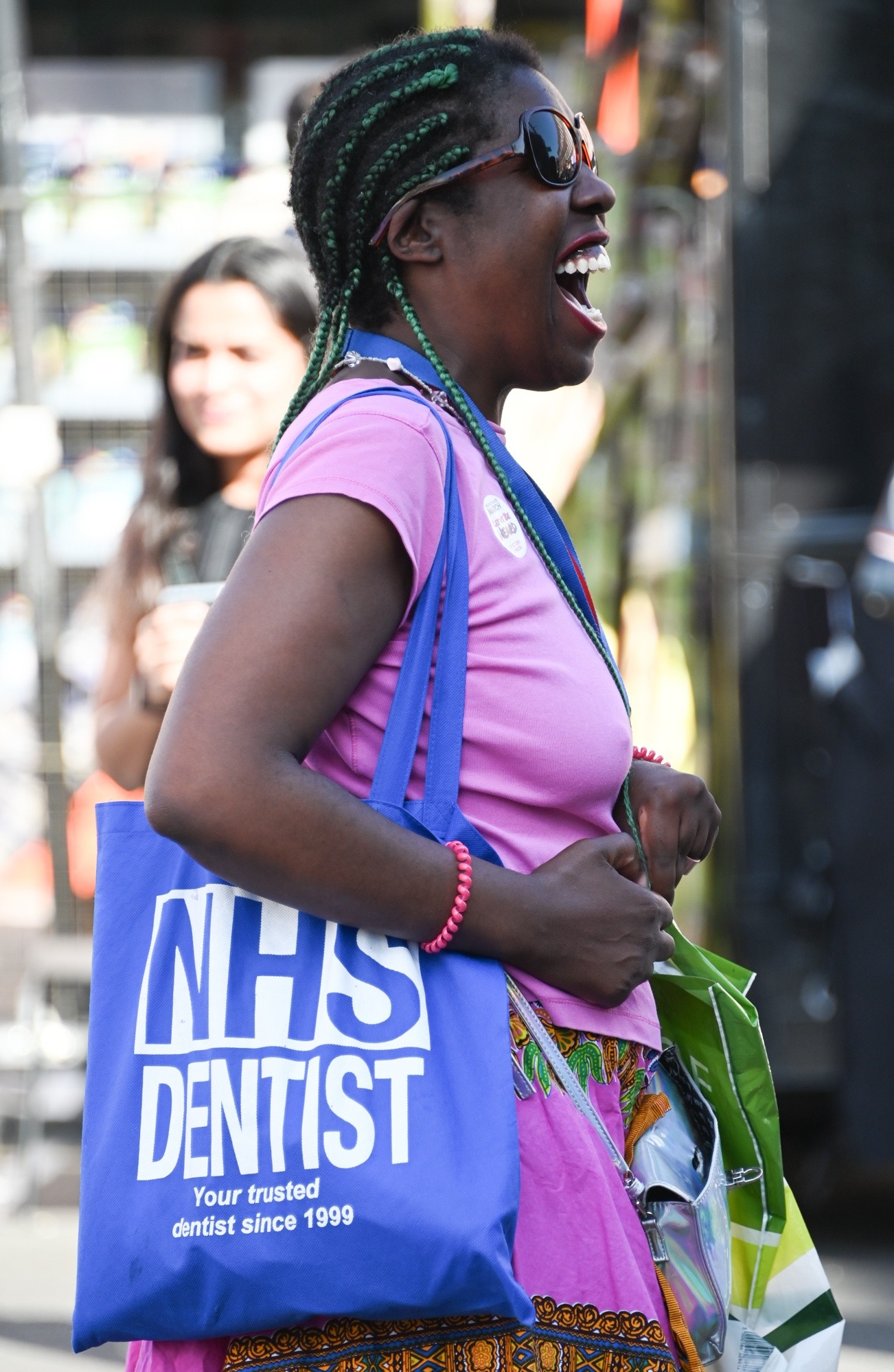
[331,349,482,450]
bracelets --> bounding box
[423,835,472,960]
[140,695,167,717]
[628,741,674,765]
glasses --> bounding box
[367,106,593,247]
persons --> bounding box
[120,30,723,1372]
[94,237,323,789]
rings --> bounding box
[686,856,701,867]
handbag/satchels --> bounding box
[74,385,535,1354]
[648,919,849,1372]
[625,1046,732,1366]
